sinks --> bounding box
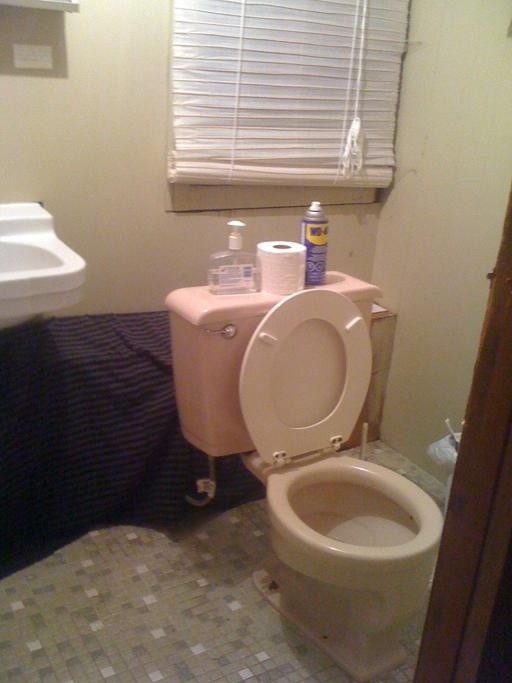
[0,202,88,317]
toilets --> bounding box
[162,267,446,681]
